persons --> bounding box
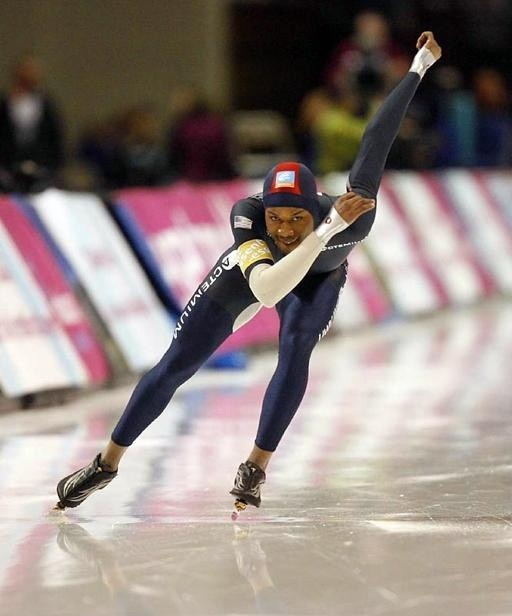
[0,43,65,194]
[55,518,289,615]
[57,31,448,506]
[301,13,512,174]
[75,74,241,195]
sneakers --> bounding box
[228,460,266,509]
[56,452,117,509]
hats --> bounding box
[263,161,318,219]
